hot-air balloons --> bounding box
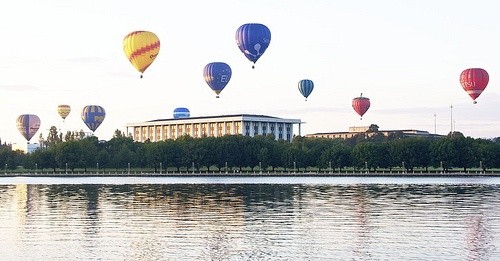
[459,68,489,105]
[123,30,160,80]
[173,108,190,119]
[57,105,70,122]
[203,62,232,99]
[297,80,314,102]
[353,97,370,120]
[16,114,40,145]
[235,23,271,69]
[82,105,105,135]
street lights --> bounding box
[449,105,453,135]
[433,114,436,134]
